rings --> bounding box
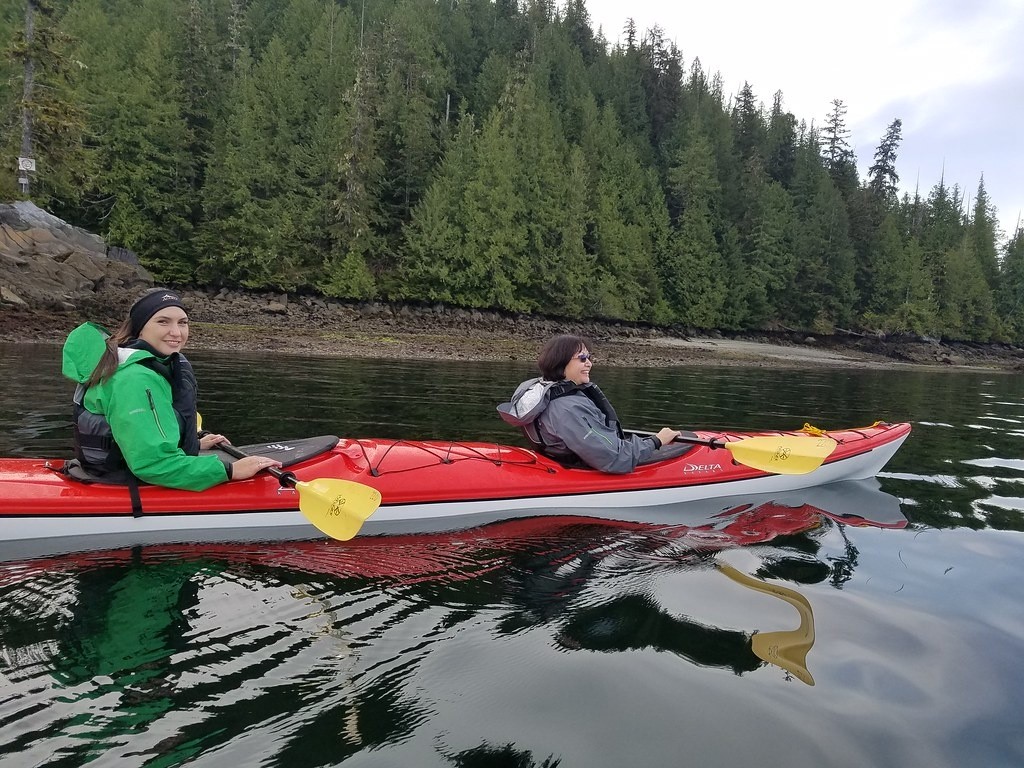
[258,460,263,465]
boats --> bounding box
[0,421,910,542]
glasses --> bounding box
[570,354,591,363]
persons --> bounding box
[74,286,283,492]
[511,334,682,474]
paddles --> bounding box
[195,412,383,542]
[620,422,841,474]
